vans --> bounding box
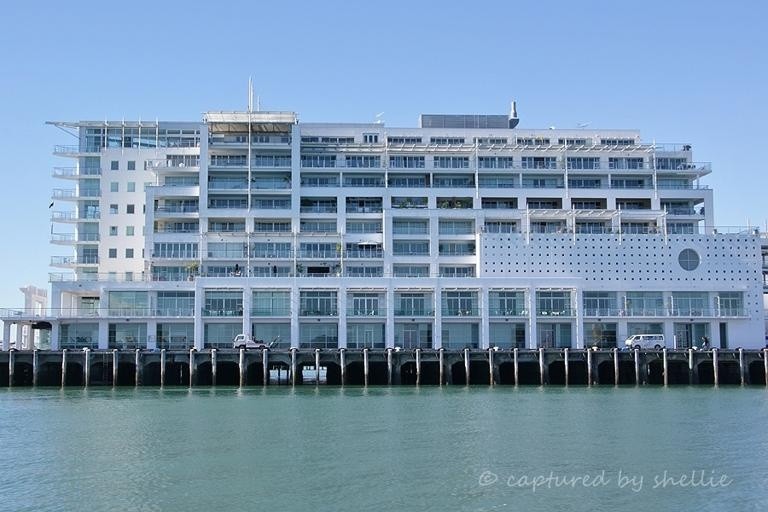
[625,334,665,350]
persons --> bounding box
[700,336,710,351]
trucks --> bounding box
[233,334,279,350]
